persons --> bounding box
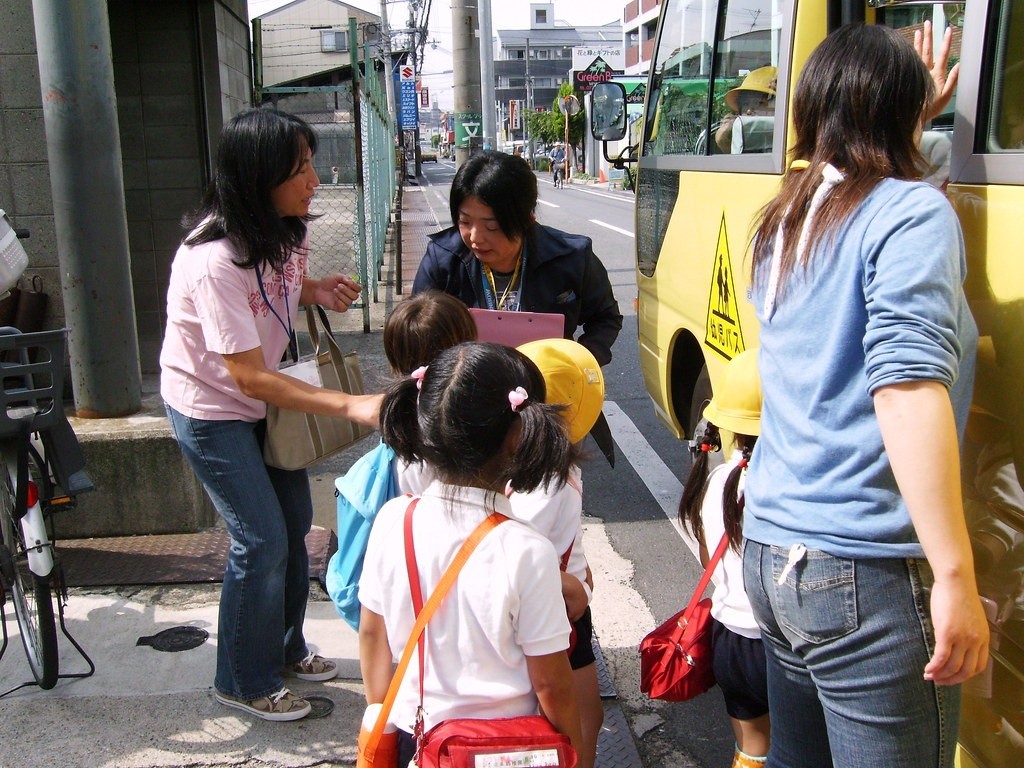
[677,347,769,768]
[159,106,387,719]
[742,19,991,768]
[331,167,339,183]
[513,147,521,155]
[410,149,623,471]
[715,67,778,154]
[550,144,568,187]
[328,290,604,768]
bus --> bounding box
[593,0,1024,552]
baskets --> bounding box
[555,161,565,169]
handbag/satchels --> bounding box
[260,304,376,472]
[324,437,397,633]
[414,715,579,768]
[638,599,714,702]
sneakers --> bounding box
[214,686,312,721]
[285,651,337,681]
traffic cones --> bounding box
[598,170,607,183]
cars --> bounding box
[420,138,455,164]
[498,139,574,172]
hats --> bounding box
[553,142,564,146]
[703,346,761,437]
[517,338,603,447]
[724,66,776,114]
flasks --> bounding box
[356,703,399,768]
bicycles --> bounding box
[0,227,96,698]
[551,157,567,189]
